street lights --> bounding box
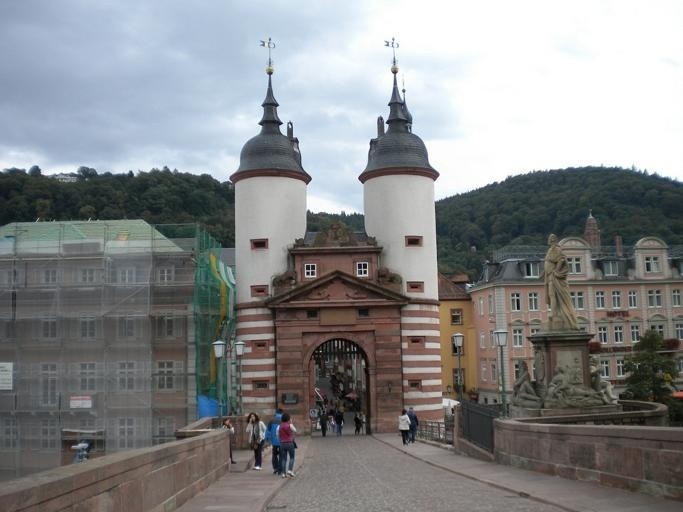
[453,332,465,402]
[235,340,245,417]
[493,329,509,419]
[213,340,226,429]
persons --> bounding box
[537,232,571,324]
[244,411,267,470]
[314,392,366,437]
[263,408,283,475]
[509,354,618,406]
[397,408,411,446]
[275,413,297,479]
[221,417,236,464]
[406,406,419,444]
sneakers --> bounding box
[252,465,262,470]
[273,469,294,479]
